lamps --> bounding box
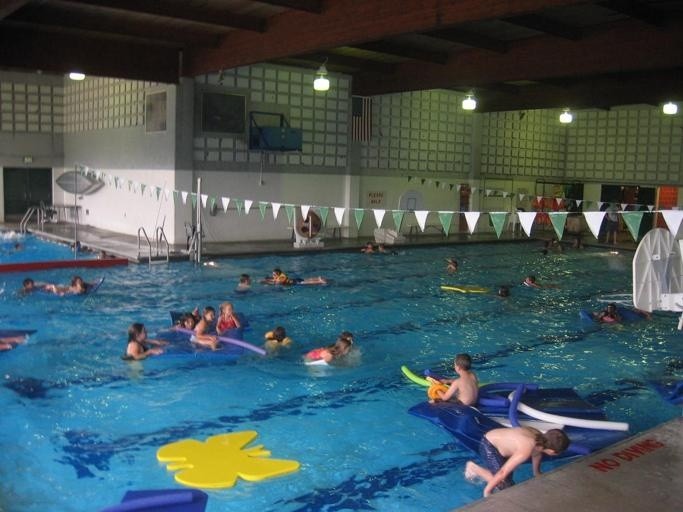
[462,89,477,110]
[663,101,677,114]
[559,107,572,123]
[314,55,330,91]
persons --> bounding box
[166,300,354,367]
[493,286,508,298]
[356,240,375,254]
[20,276,99,296]
[256,268,330,285]
[523,276,558,289]
[447,260,458,272]
[377,242,396,255]
[237,273,253,289]
[71,240,95,255]
[594,304,621,323]
[124,323,172,360]
[460,425,569,498]
[430,353,479,407]
[14,244,21,250]
[0,336,25,352]
[540,237,584,256]
[95,248,141,264]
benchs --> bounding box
[287,225,347,242]
[50,205,82,224]
[408,223,453,239]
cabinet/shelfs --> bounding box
[478,173,677,248]
